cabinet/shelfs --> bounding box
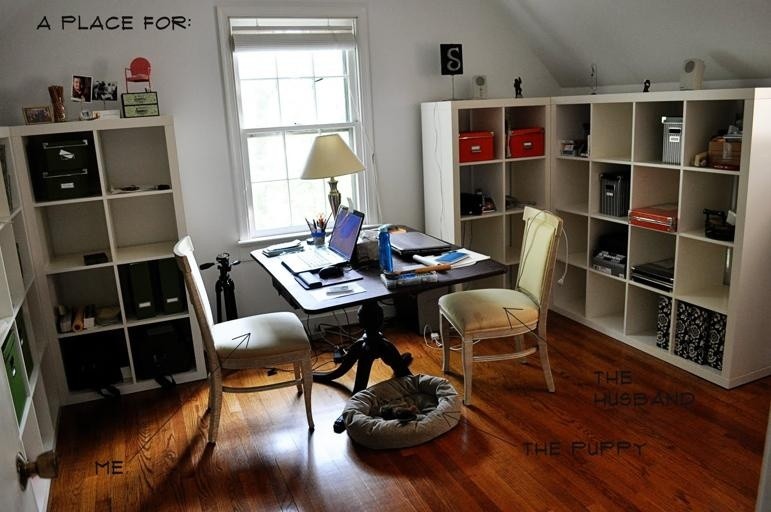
[419,95,550,293]
[9,112,209,410]
[552,86,770,391]
[0,125,61,512]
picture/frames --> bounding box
[20,103,55,126]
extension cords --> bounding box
[305,327,327,339]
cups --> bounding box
[312,232,325,245]
[48,86,68,122]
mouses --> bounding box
[319,265,344,279]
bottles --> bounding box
[378,227,393,277]
[475,189,486,206]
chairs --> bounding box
[122,56,152,93]
[171,235,317,446]
[434,205,565,407]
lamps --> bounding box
[298,133,367,221]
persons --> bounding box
[73,76,92,102]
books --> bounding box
[260,238,304,258]
[411,247,492,271]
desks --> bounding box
[248,222,510,433]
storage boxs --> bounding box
[23,131,101,203]
[598,171,629,217]
[14,306,33,381]
[661,114,683,165]
[458,130,496,162]
[1,328,26,427]
[506,126,544,160]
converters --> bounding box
[334,352,345,363]
[430,332,440,341]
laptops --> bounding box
[389,231,457,256]
[281,204,365,276]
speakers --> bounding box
[473,74,489,98]
[680,57,705,91]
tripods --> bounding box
[199,252,242,323]
